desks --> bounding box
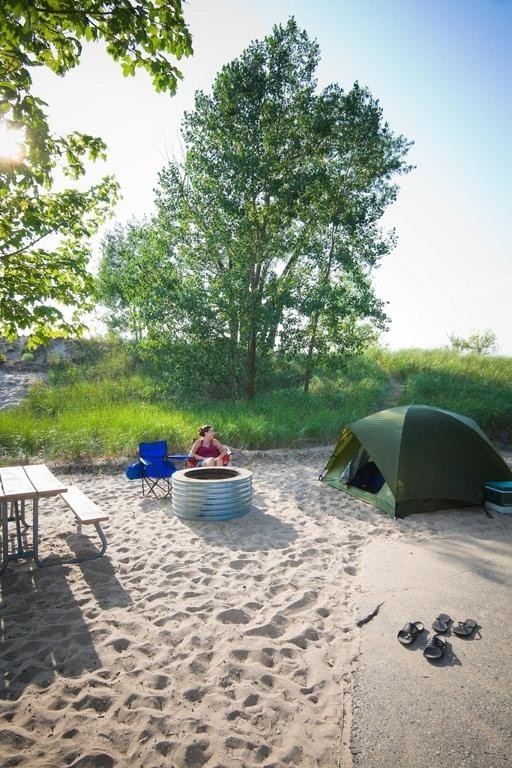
[0,464,68,577]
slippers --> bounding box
[432,614,451,632]
[398,622,423,644]
[453,619,476,635]
[423,633,446,658]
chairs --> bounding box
[185,434,236,468]
[126,440,177,500]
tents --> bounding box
[317,401,512,520]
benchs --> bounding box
[59,486,108,558]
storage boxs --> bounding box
[483,481,512,514]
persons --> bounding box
[190,425,227,467]
